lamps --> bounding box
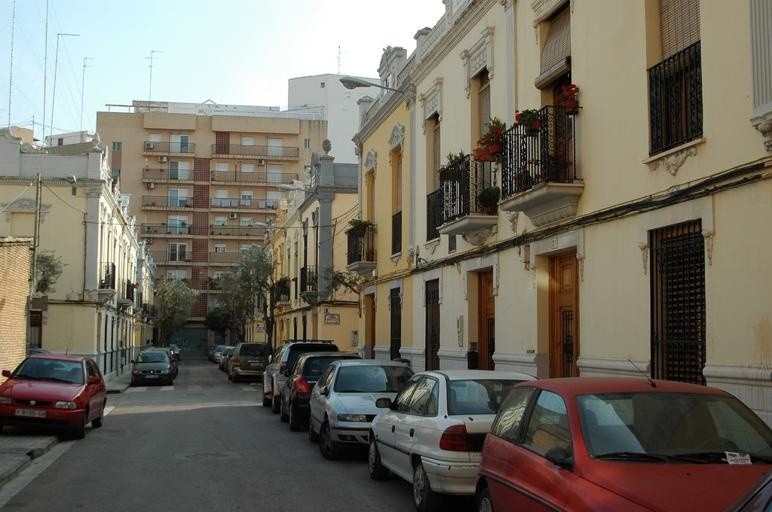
[339,76,416,108]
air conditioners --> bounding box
[257,159,264,166]
[229,212,237,219]
[144,141,153,149]
[145,182,155,189]
[158,155,169,164]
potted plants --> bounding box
[478,184,500,216]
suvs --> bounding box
[226,342,274,383]
[259,339,339,413]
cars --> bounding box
[131,342,182,384]
[280,350,365,426]
[473,373,772,512]
[308,359,416,459]
[209,345,235,369]
[368,370,562,511]
[0,353,107,439]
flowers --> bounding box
[526,143,573,167]
[476,133,491,154]
[560,82,578,100]
[510,164,532,181]
[487,115,506,142]
[514,109,543,130]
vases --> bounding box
[473,149,496,162]
[525,129,538,136]
[542,167,559,181]
[489,142,502,153]
[516,181,528,191]
[560,101,577,115]
